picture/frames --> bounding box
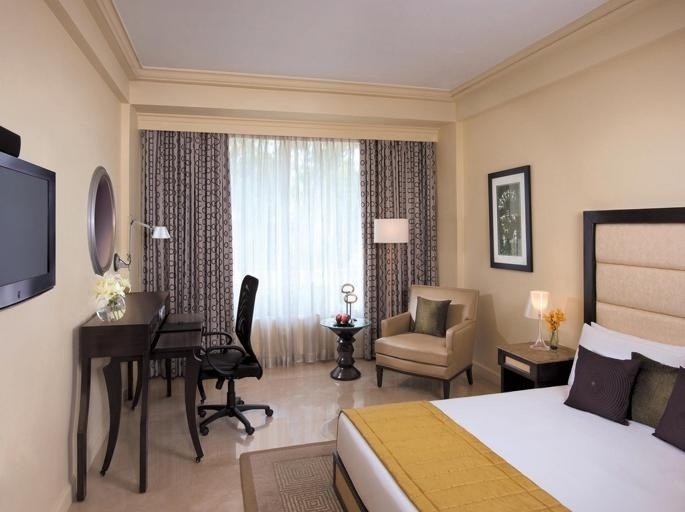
[487,165,536,271]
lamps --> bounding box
[522,289,550,350]
[372,218,409,319]
[115,219,172,269]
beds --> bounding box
[330,204,685,512]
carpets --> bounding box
[239,438,345,511]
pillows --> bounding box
[652,367,684,450]
[563,344,645,429]
[414,296,452,337]
[565,324,683,413]
[630,349,683,428]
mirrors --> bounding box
[85,164,118,275]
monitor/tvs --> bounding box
[1,150,59,310]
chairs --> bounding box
[373,284,482,398]
[196,275,273,438]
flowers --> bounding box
[542,307,566,330]
[95,272,131,300]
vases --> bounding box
[92,297,129,320]
[542,331,566,350]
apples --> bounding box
[336,314,342,321]
[342,314,349,323]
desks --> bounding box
[72,290,173,502]
[101,314,204,478]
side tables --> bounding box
[319,316,372,381]
